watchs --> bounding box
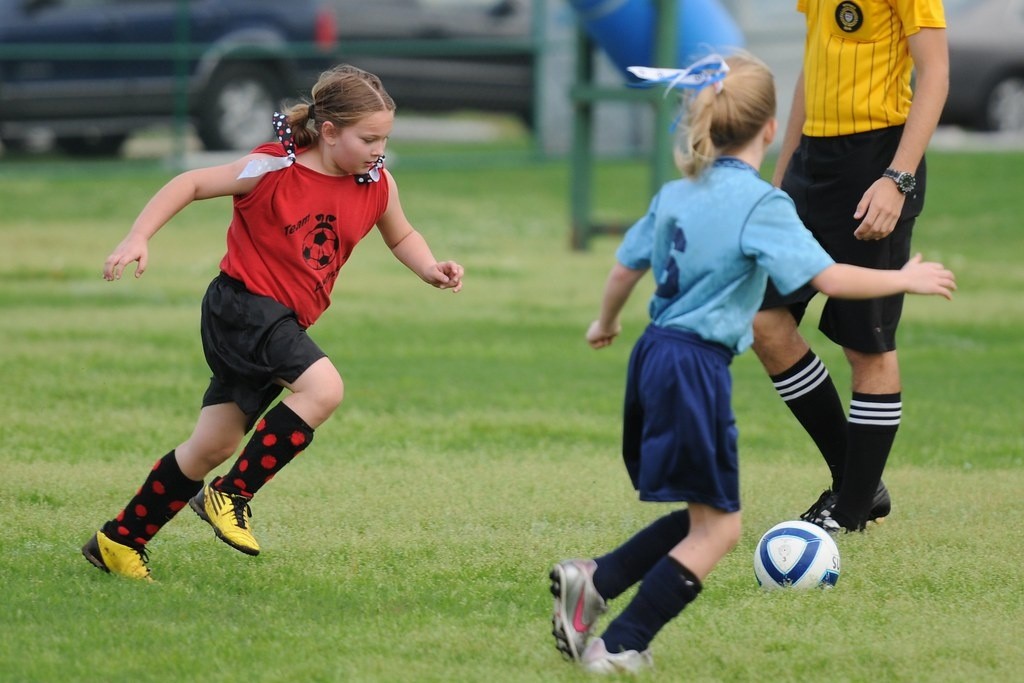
[882,167,917,199]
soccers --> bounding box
[754,521,840,591]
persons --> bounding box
[78,63,464,587]
[549,54,958,679]
[744,2,955,538]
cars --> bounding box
[912,0,1024,137]
[0,0,531,158]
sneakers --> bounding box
[549,559,609,664]
[82,530,160,584]
[582,638,654,678]
[189,476,260,556]
[800,479,891,531]
